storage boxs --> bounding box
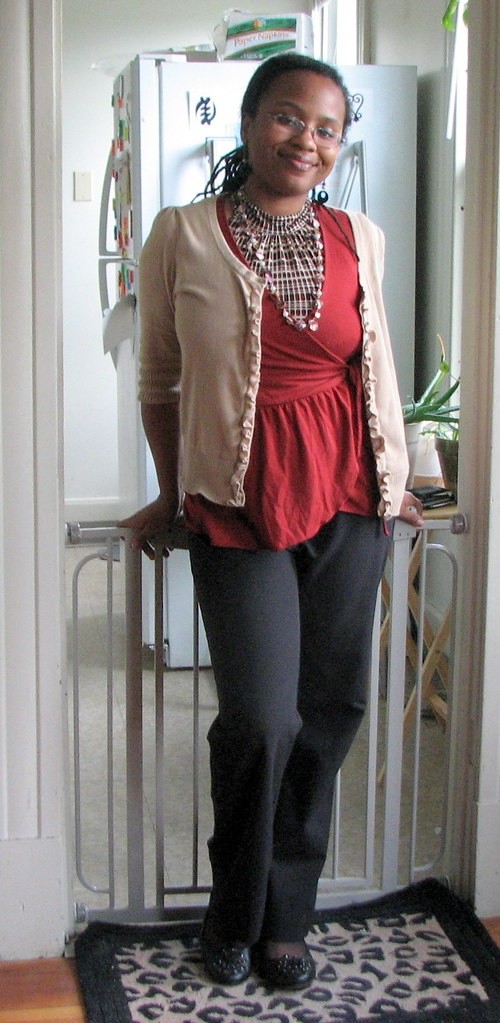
[224,12,316,61]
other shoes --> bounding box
[201,927,251,984]
[260,939,316,989]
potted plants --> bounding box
[403,336,460,498]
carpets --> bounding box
[73,877,500,1023]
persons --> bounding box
[117,53,423,990]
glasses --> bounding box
[256,110,347,149]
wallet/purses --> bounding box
[408,485,456,509]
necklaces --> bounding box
[228,186,325,332]
